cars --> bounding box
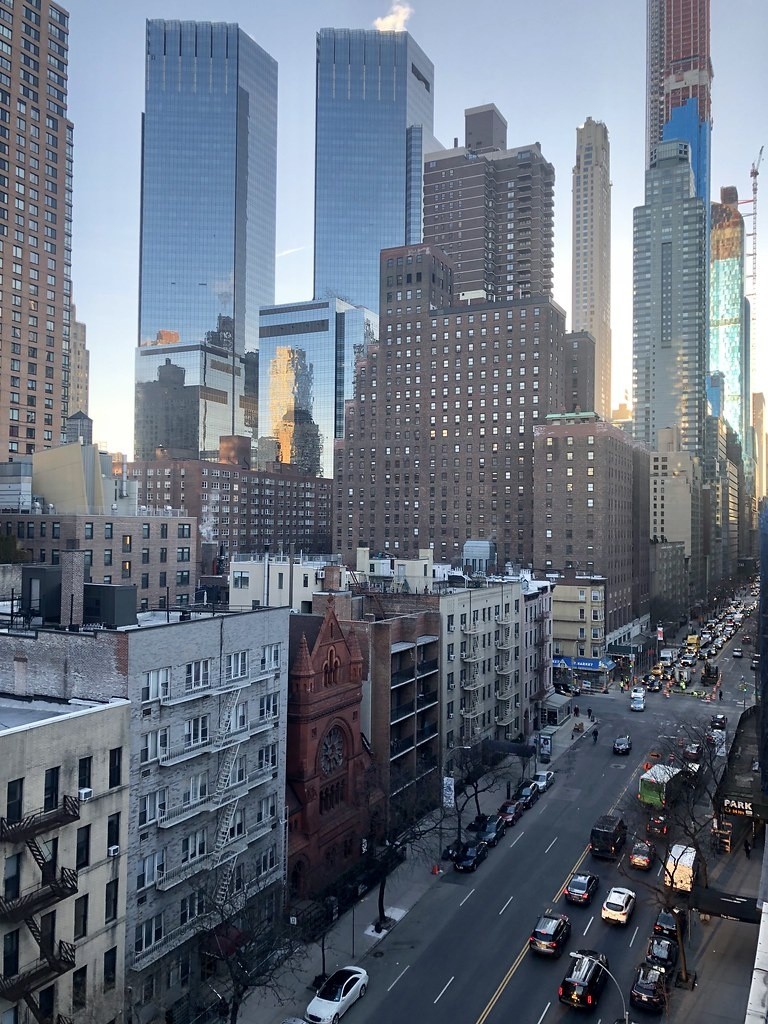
[631,907,686,1014]
[683,742,704,761]
[565,871,599,906]
[630,566,761,743]
[304,965,369,1024]
[454,770,556,874]
[630,841,656,872]
[613,735,632,755]
[647,814,674,840]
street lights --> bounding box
[352,900,364,959]
[570,952,629,1024]
[569,641,579,715]
[438,746,471,861]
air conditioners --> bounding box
[460,625,466,630]
[449,770,454,777]
[449,742,453,747]
[460,653,467,658]
[449,713,454,718]
[474,728,480,734]
[516,703,521,707]
[495,641,499,645]
[495,717,499,722]
[515,610,518,613]
[451,684,455,689]
[450,626,456,631]
[515,672,518,676]
[460,710,464,715]
[495,665,501,671]
[515,633,519,637]
[108,845,120,857]
[506,733,512,739]
[450,655,456,660]
[517,656,519,660]
[462,681,467,687]
[79,788,93,801]
[495,615,500,621]
[530,666,535,670]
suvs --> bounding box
[602,887,637,928]
[530,914,571,960]
[558,949,610,1014]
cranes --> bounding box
[750,145,765,320]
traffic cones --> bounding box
[692,668,696,673]
[666,693,670,698]
[711,693,717,700]
[706,696,710,702]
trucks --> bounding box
[665,844,698,892]
[590,816,628,863]
[638,764,685,812]
